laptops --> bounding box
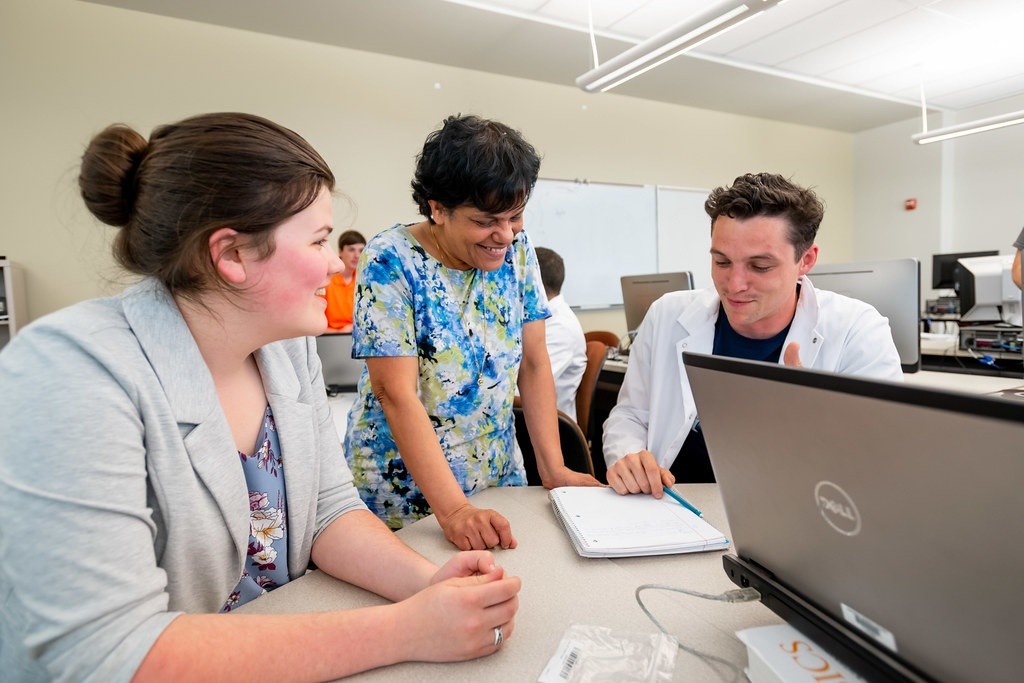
[314,333,363,394]
[681,348,1024,683]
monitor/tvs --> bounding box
[807,251,1024,374]
[621,271,695,343]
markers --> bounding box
[609,303,626,306]
[569,306,582,309]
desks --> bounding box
[228,482,782,683]
[905,370,1024,400]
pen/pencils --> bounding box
[661,484,706,519]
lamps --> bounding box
[576,0,785,94]
[910,75,1024,146]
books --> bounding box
[546,482,732,559]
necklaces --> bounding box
[435,228,487,386]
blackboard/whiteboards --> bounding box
[655,185,714,290]
[522,177,658,311]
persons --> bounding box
[0,113,522,683]
[512,247,588,424]
[601,172,903,499]
[342,112,611,553]
[321,230,366,329]
[1011,226,1024,290]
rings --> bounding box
[493,626,503,646]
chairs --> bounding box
[584,330,620,396]
[513,406,595,486]
[575,341,609,437]
